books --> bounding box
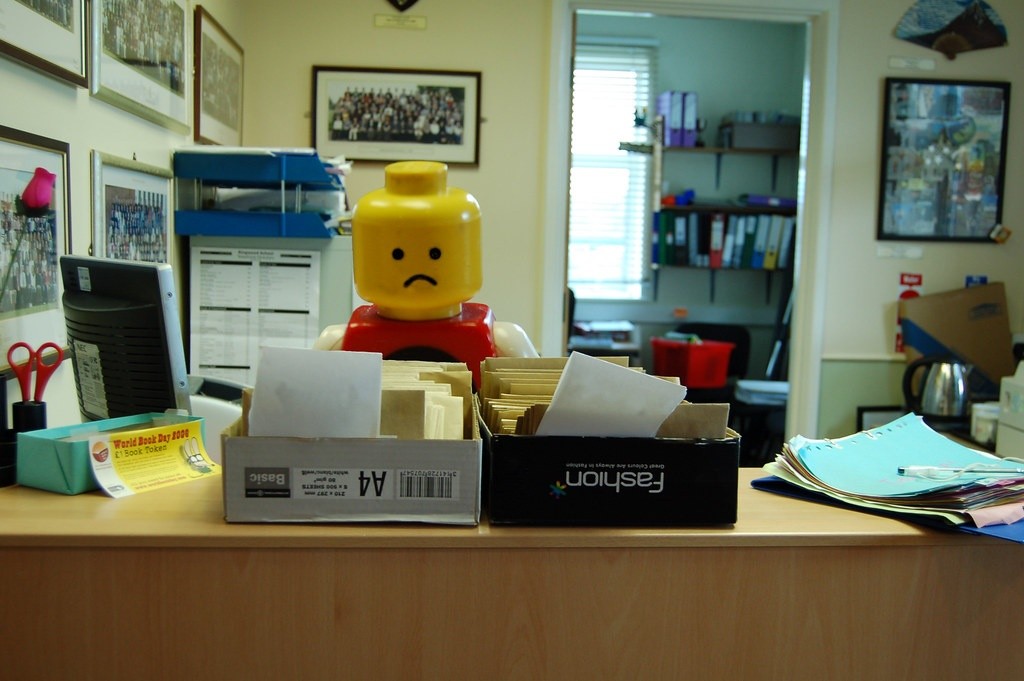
[734,378,1024,528]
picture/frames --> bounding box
[857,405,905,433]
[85,148,177,283]
[1,0,94,92]
[310,64,484,169]
[87,2,194,141]
[189,4,248,153]
[0,122,73,311]
[874,75,1014,245]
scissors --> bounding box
[7,342,64,404]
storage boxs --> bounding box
[218,363,488,528]
[651,337,736,390]
[717,120,799,150]
[471,354,742,530]
[899,278,1015,399]
[16,411,207,495]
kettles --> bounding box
[902,352,977,431]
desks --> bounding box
[1,466,1024,680]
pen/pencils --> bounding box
[898,467,1023,479]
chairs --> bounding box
[677,321,752,429]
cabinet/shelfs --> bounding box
[650,113,796,269]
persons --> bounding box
[20,0,240,128]
[105,200,168,264]
[0,201,59,311]
[330,84,464,145]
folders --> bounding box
[682,91,698,148]
[656,90,682,147]
[660,212,795,270]
[747,194,797,208]
